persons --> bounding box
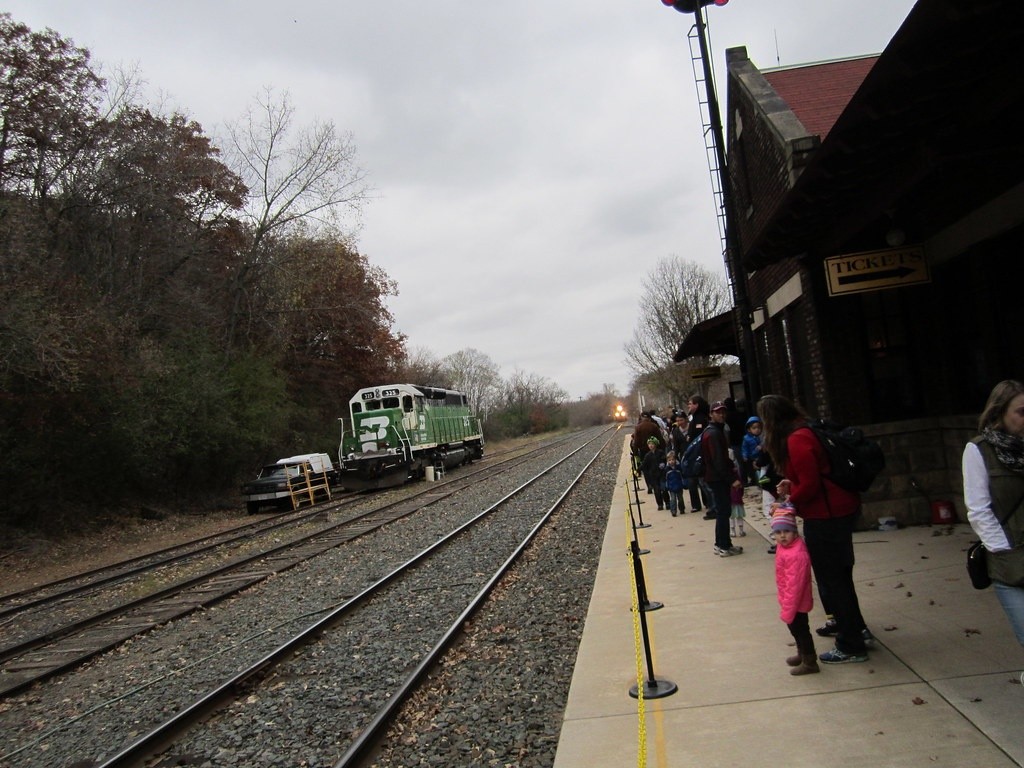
[962,379,1024,687]
[641,437,671,512]
[673,410,712,512]
[660,449,685,517]
[815,576,838,637]
[630,405,676,495]
[741,416,764,465]
[701,398,746,557]
[686,396,710,445]
[768,509,822,676]
[758,395,874,663]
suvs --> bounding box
[241,453,339,515]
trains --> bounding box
[338,384,486,486]
[614,403,628,423]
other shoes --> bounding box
[672,511,676,516]
[666,504,670,509]
[704,511,716,519]
[680,509,685,514]
[691,507,700,513]
[658,505,664,510]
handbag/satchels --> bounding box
[966,541,994,590]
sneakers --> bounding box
[730,531,746,538]
[818,649,868,664]
[767,543,777,554]
[862,630,874,648]
[815,618,838,636]
[713,546,743,558]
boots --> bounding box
[786,648,820,676]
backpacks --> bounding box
[796,416,885,494]
[681,426,722,480]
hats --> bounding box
[710,401,728,412]
[771,502,798,534]
[647,436,660,446]
